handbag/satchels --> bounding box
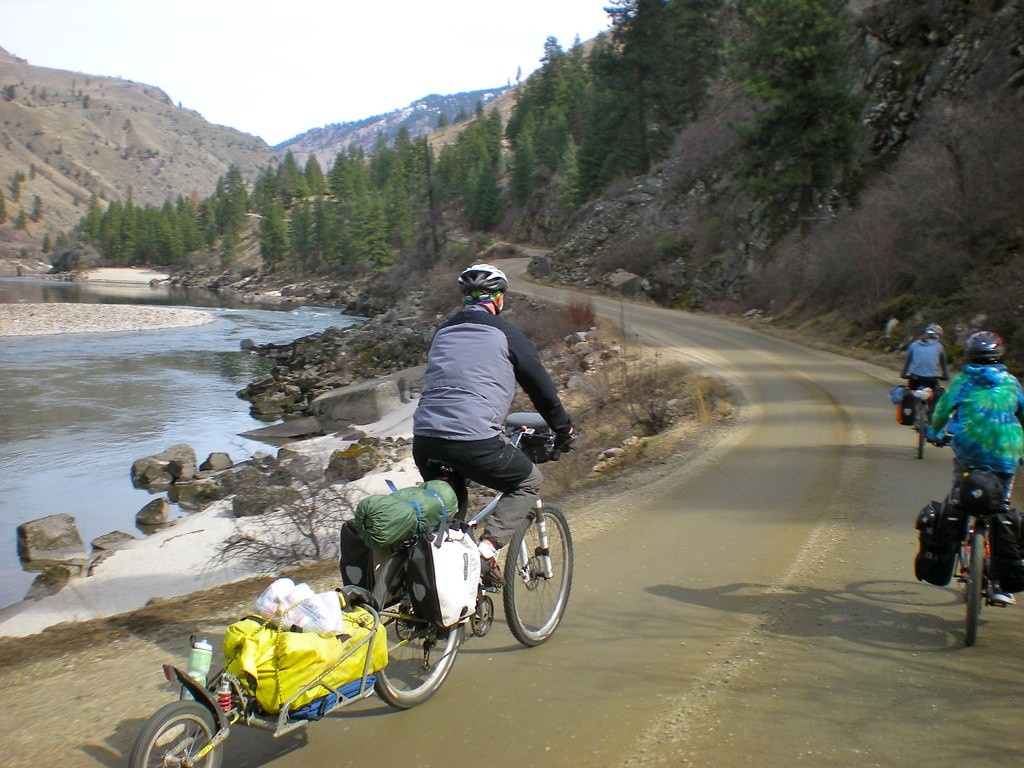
[340,519,406,611]
[991,508,1024,593]
[957,465,1008,516]
[403,518,481,625]
[889,386,916,426]
[927,388,949,425]
[915,487,967,587]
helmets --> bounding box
[926,324,943,337]
[459,263,508,294]
[963,331,1005,363]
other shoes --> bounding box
[990,587,1016,605]
[960,566,969,578]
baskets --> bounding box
[505,409,554,464]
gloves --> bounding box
[553,425,578,454]
[926,426,946,439]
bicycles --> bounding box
[910,383,936,460]
[367,408,573,711]
[943,435,1007,645]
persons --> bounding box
[926,331,1023,604]
[901,323,951,425]
[412,263,574,588]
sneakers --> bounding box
[479,550,506,588]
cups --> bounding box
[181,638,212,700]
[260,579,343,637]
